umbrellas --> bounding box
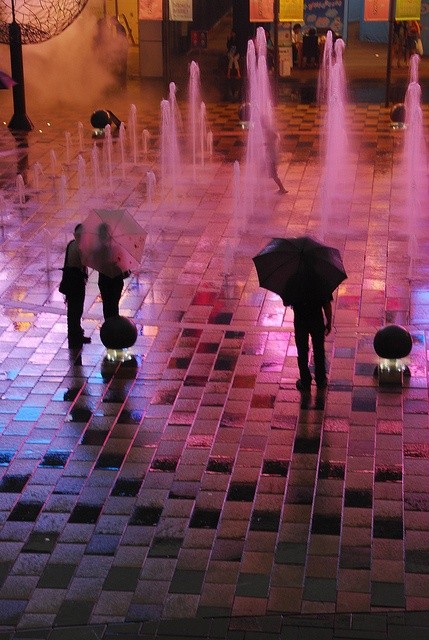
[73,208,147,277]
[251,237,348,302]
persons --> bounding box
[92,19,106,47]
[95,223,135,322]
[111,15,127,39]
[226,32,242,81]
[290,23,301,67]
[280,292,334,390]
[265,29,274,79]
[262,123,289,195]
[392,17,404,70]
[404,19,420,67]
[59,224,91,349]
